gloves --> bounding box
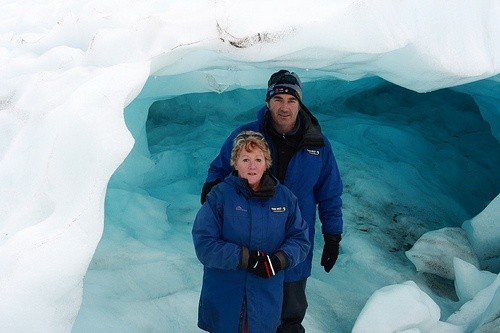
[246,250,281,279]
[321,239,340,273]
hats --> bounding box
[265,70,303,104]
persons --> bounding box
[192,131,311,333]
[201,69,344,333]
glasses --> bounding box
[235,133,265,142]
